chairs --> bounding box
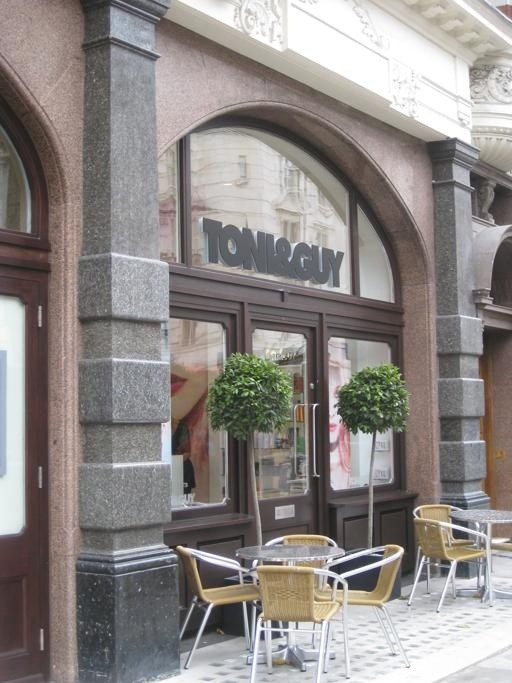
[250,565,351,682]
[176,546,256,670]
[311,545,410,679]
[408,505,491,612]
[252,534,338,648]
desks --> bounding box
[235,545,345,673]
[448,507,510,602]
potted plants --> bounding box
[333,365,410,604]
[205,352,296,642]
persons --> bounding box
[325,337,351,491]
[170,318,209,506]
